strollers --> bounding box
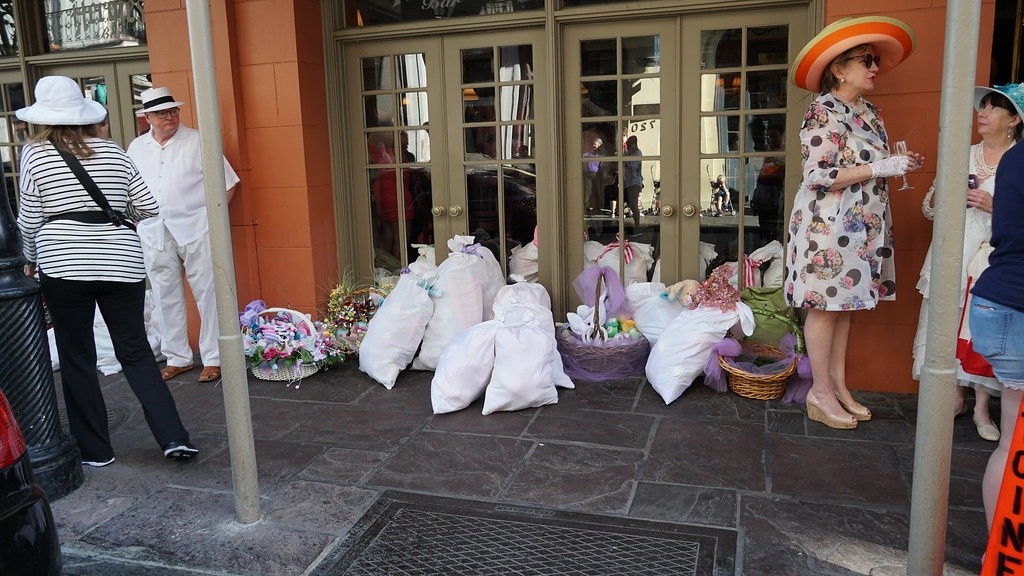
[707,164,738,217]
[650,165,661,216]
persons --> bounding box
[125,86,240,379]
[368,125,417,260]
[783,14,916,430]
[713,175,730,216]
[512,145,535,173]
[624,136,643,237]
[583,137,609,214]
[912,82,1024,565]
[11,76,199,466]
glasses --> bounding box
[836,54,880,69]
[150,107,180,119]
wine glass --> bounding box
[893,140,915,191]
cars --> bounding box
[412,164,536,247]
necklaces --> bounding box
[974,140,997,180]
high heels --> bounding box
[834,393,872,421]
[806,387,857,429]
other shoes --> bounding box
[953,403,965,419]
[973,413,1001,441]
[164,440,198,461]
[80,456,115,467]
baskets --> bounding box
[555,269,650,377]
[244,308,322,381]
[719,308,804,400]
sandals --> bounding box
[160,361,195,381]
[198,366,222,381]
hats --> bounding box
[135,86,185,114]
[14,76,107,125]
[973,83,1024,139]
[790,16,917,95]
